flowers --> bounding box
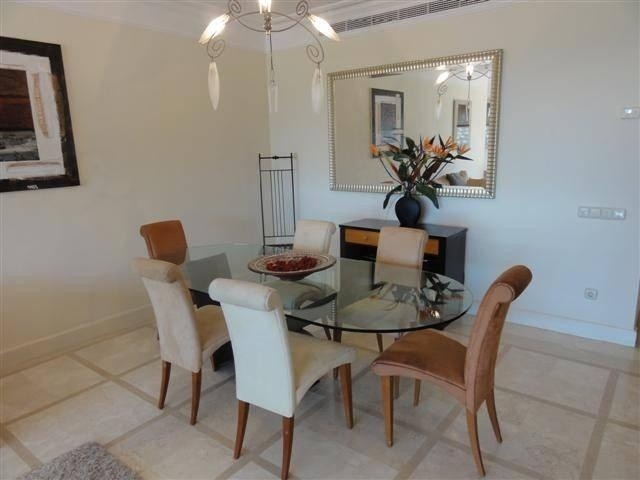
[369,133,470,209]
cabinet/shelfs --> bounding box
[339,219,467,330]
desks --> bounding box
[174,241,475,333]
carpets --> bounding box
[11,442,143,479]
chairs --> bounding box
[208,277,361,480]
[369,264,532,477]
[292,219,338,257]
[140,220,187,265]
[130,258,230,424]
[374,225,429,351]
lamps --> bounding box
[430,60,493,118]
[200,0,341,115]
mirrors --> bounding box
[321,50,504,200]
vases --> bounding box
[394,192,423,227]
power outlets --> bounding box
[590,207,602,218]
[616,208,626,220]
[621,106,638,118]
[603,208,614,221]
[577,207,589,217]
[585,288,598,300]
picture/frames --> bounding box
[0,37,79,193]
[450,98,473,152]
[367,87,407,159]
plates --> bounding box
[246,250,337,282]
